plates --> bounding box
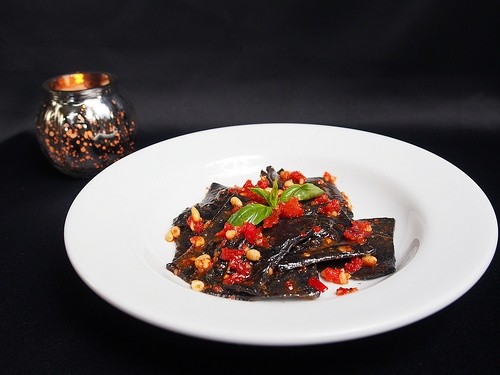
[64,124,498,346]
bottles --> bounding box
[35,70,135,177]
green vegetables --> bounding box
[227,177,324,227]
